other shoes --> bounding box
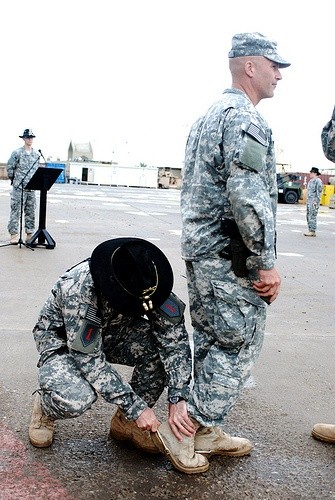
[311,423,335,443]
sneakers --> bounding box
[110,410,165,454]
[25,234,33,242]
[11,234,18,243]
[29,387,54,447]
[194,426,254,456]
[150,413,209,474]
[304,232,316,237]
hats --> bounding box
[19,129,36,138]
[228,32,291,68]
[310,167,321,175]
[90,238,174,321]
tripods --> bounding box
[0,155,41,251]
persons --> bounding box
[310,100,335,445]
[7,129,42,245]
[304,167,323,237]
[151,30,292,475]
[28,237,198,451]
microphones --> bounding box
[38,149,46,160]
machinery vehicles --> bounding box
[276,163,303,205]
